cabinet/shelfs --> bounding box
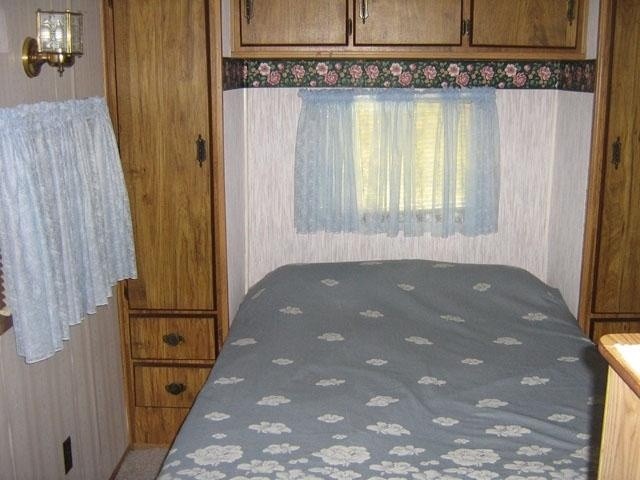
[232,0,588,61]
[578,1,640,344]
[99,1,229,447]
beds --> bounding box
[156,262,608,480]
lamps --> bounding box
[22,10,84,76]
